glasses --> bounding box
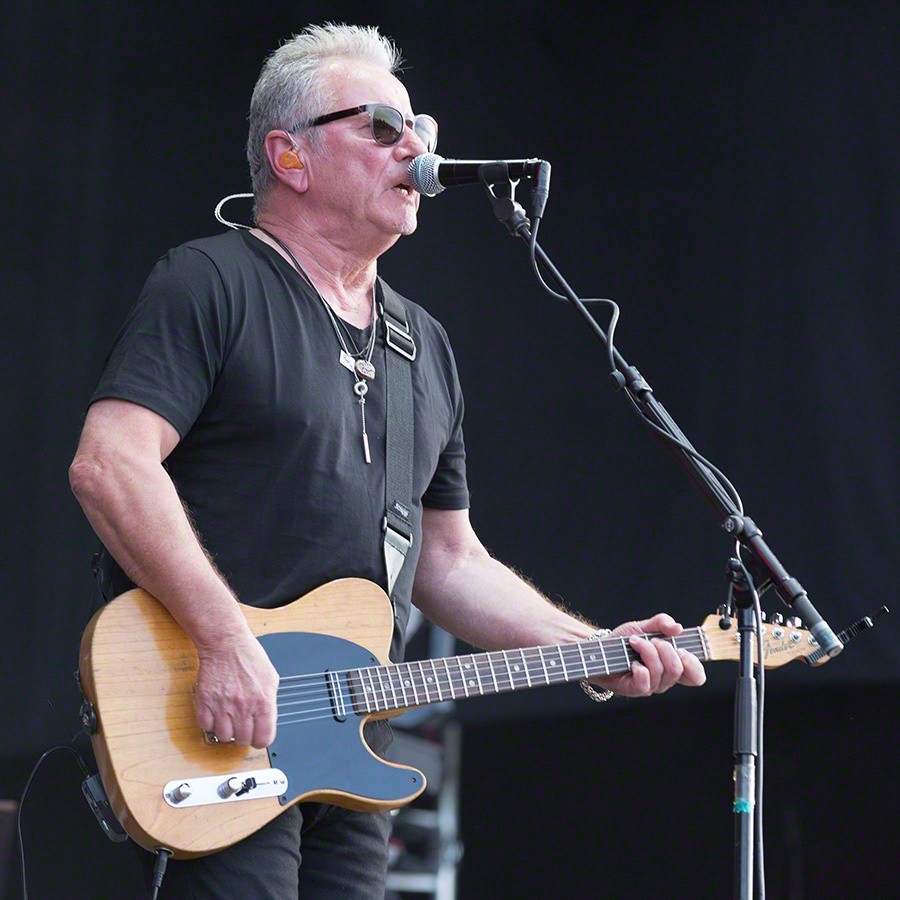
[289,103,438,153]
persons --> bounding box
[67,20,709,900]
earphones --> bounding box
[278,152,304,170]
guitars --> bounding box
[74,576,891,860]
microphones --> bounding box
[406,153,551,198]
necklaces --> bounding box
[256,220,380,467]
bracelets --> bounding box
[579,628,618,704]
